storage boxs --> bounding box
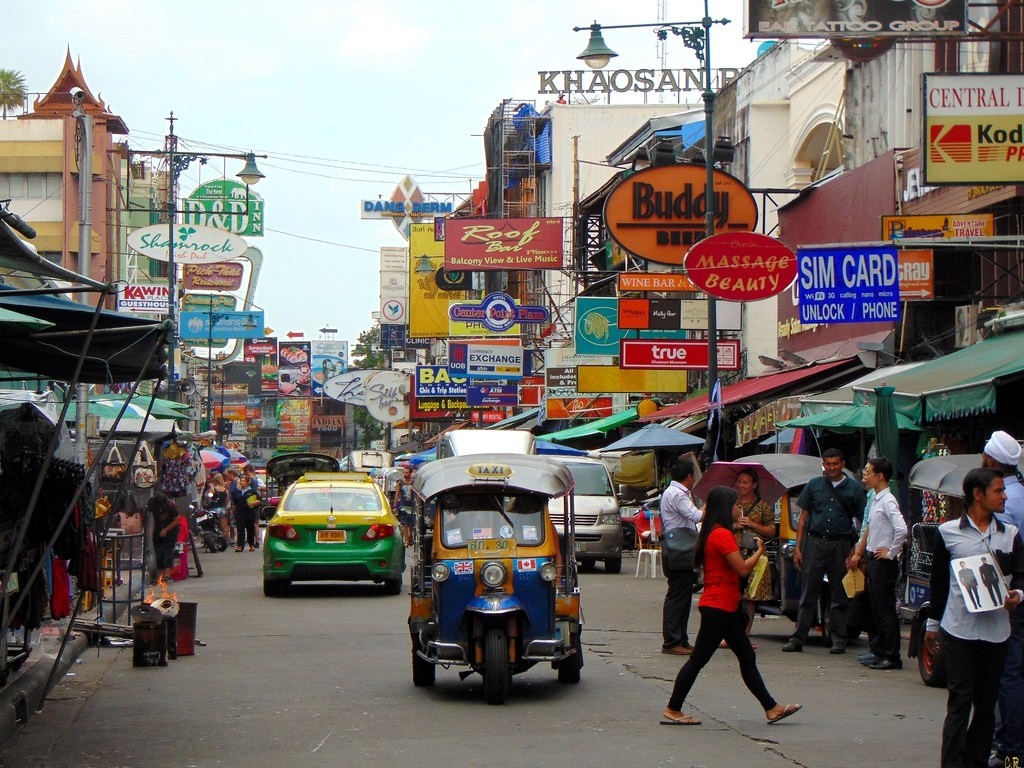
[0,570,19,596]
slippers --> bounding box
[767,703,803,724]
[659,715,702,725]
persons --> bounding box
[846,457,910,670]
[958,560,983,610]
[146,494,181,589]
[924,467,1023,768]
[780,450,871,655]
[713,467,778,649]
[658,486,805,726]
[977,428,1024,768]
[321,359,335,381]
[978,557,1006,606]
[332,361,345,377]
[183,436,272,558]
[655,459,705,658]
[392,465,415,550]
[848,460,886,666]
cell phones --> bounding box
[741,529,759,550]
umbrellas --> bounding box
[691,459,791,524]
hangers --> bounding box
[0,401,87,490]
[917,436,948,459]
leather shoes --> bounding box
[869,659,903,669]
[860,657,882,665]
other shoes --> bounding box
[235,545,244,552]
[856,652,875,660]
[829,641,847,654]
[161,575,173,583]
[254,541,260,548]
[782,642,802,652]
[661,644,695,655]
[249,545,255,552]
[987,754,1005,767]
[151,576,158,586]
[226,538,236,546]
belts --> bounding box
[809,531,852,541]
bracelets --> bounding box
[392,505,397,509]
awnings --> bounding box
[408,356,1020,521]
[56,389,196,437]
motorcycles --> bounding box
[189,492,227,553]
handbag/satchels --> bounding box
[663,527,700,571]
[225,505,231,517]
[116,493,143,536]
[130,442,158,489]
[100,446,128,484]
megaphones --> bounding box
[176,376,196,396]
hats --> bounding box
[984,430,1022,466]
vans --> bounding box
[535,454,629,574]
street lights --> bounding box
[571,12,731,464]
[160,113,266,406]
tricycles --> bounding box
[726,454,869,642]
[910,455,984,686]
[409,452,586,703]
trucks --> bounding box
[339,450,416,513]
[436,428,535,456]
[264,453,340,522]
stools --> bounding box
[81,590,92,612]
[635,548,664,579]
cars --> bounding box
[262,471,412,596]
